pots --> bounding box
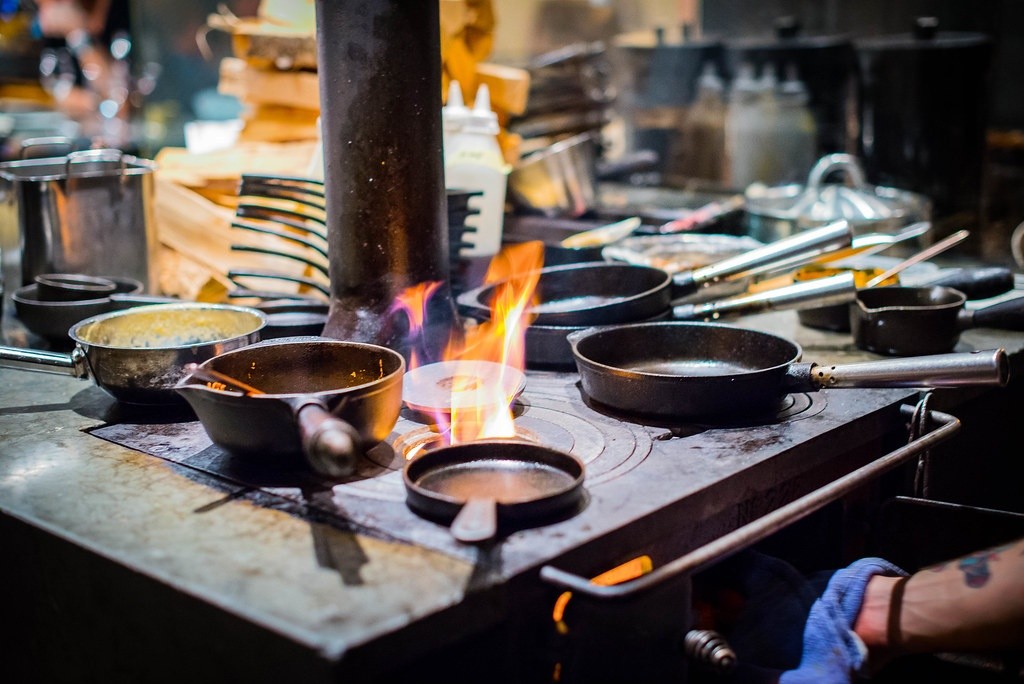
[173,336,405,475]
[745,153,933,263]
[0,137,156,343]
[404,438,586,543]
[851,285,977,358]
[457,218,855,368]
[566,320,1010,420]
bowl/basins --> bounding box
[68,302,268,405]
[12,273,144,343]
[507,133,599,222]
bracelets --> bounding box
[71,36,99,56]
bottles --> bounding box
[681,66,724,184]
[303,115,327,292]
[442,80,507,290]
[724,63,817,192]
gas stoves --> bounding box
[87,317,962,600]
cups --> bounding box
[183,120,241,153]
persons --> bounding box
[1,0,200,160]
[845,540,1024,662]
